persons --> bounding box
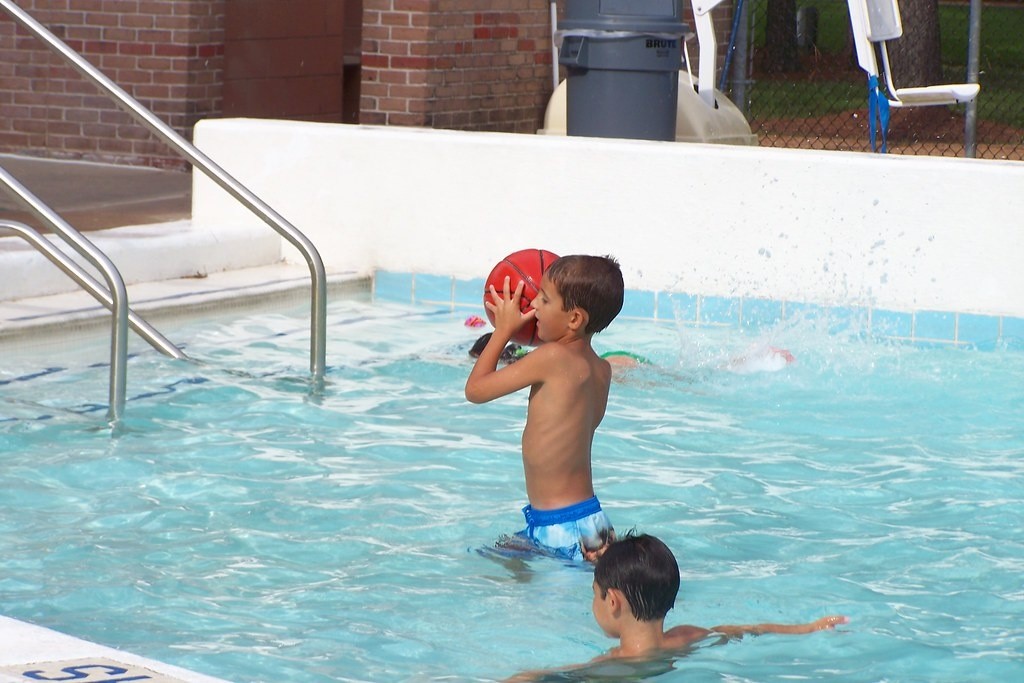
[468,325,793,383]
[465,252,625,565]
[507,530,851,682]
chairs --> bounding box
[846,0,981,152]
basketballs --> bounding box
[484,249,562,347]
